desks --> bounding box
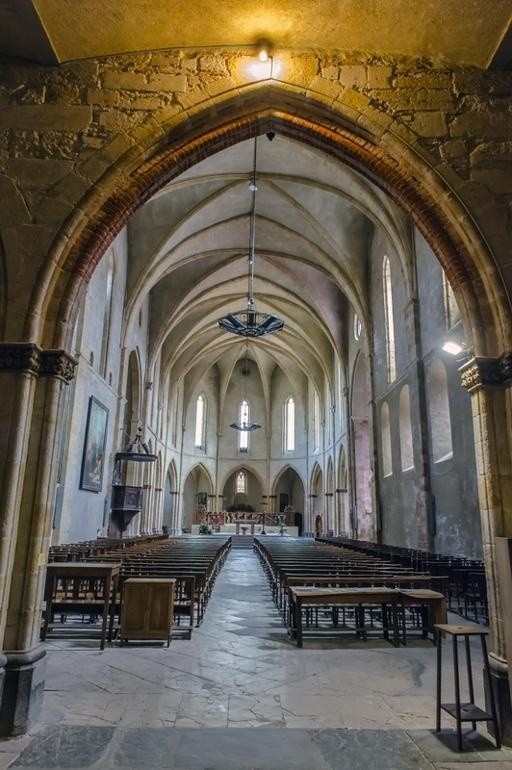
[234,520,257,535]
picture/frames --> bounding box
[79,395,109,491]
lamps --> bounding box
[442,336,471,360]
[256,49,270,65]
[218,135,286,336]
[230,341,262,431]
[111,426,157,535]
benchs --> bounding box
[250,533,485,648]
[44,534,231,648]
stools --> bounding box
[433,624,501,752]
